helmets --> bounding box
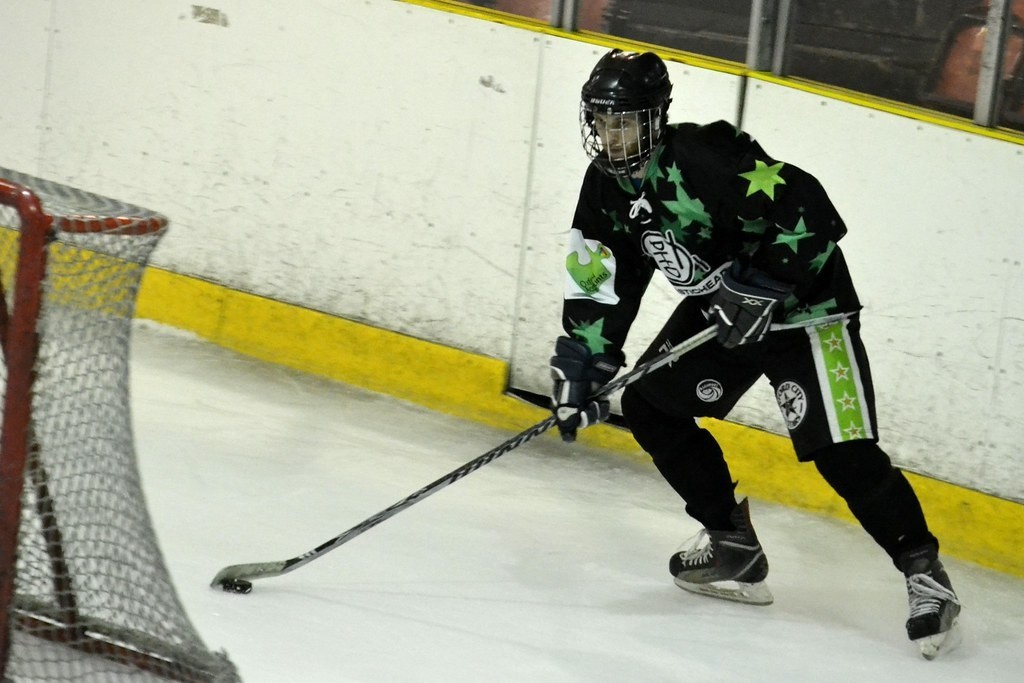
[579,48,673,178]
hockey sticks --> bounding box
[208,320,726,588]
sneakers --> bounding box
[669,496,774,605]
[893,535,965,662]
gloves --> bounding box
[709,255,790,348]
[551,336,626,442]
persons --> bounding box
[552,48,965,661]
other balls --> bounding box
[220,577,253,593]
